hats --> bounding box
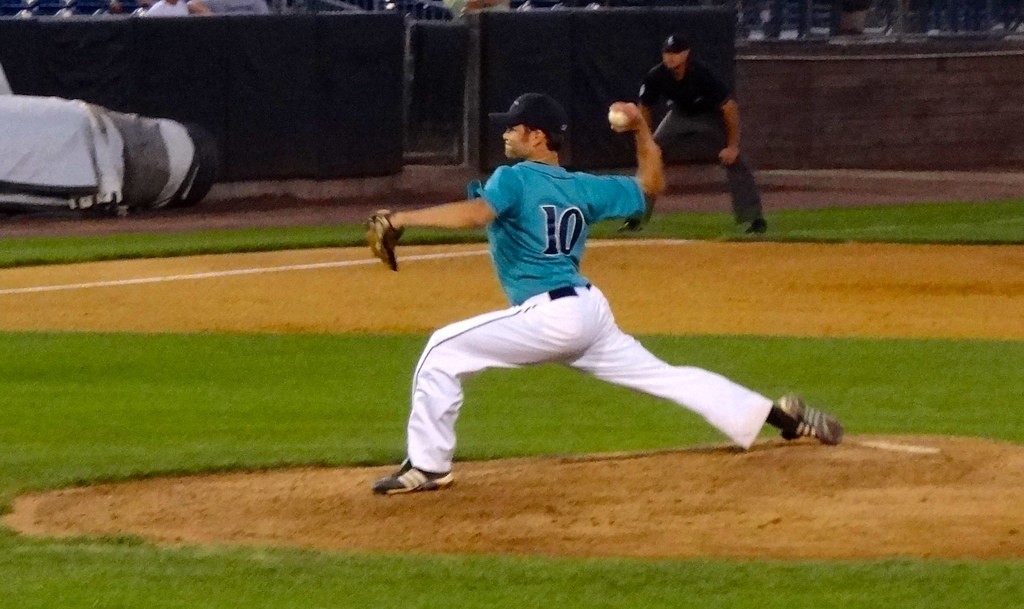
[662,34,690,53]
[487,92,572,137]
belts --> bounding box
[521,283,593,312]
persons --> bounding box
[613,31,768,233]
[362,94,845,499]
[0,1,283,20]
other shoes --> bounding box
[618,219,642,232]
[747,219,767,232]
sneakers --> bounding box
[771,392,845,445]
[373,458,454,493]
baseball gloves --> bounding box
[365,208,406,272]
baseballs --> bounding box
[609,108,630,128]
